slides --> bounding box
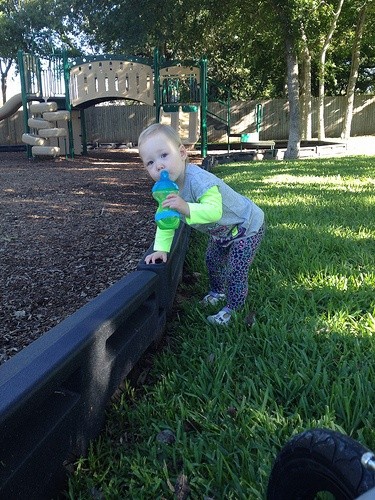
[0,93,22,120]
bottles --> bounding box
[151,171,181,231]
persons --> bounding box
[137,123,265,324]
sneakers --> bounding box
[200,292,226,306]
[207,307,233,326]
